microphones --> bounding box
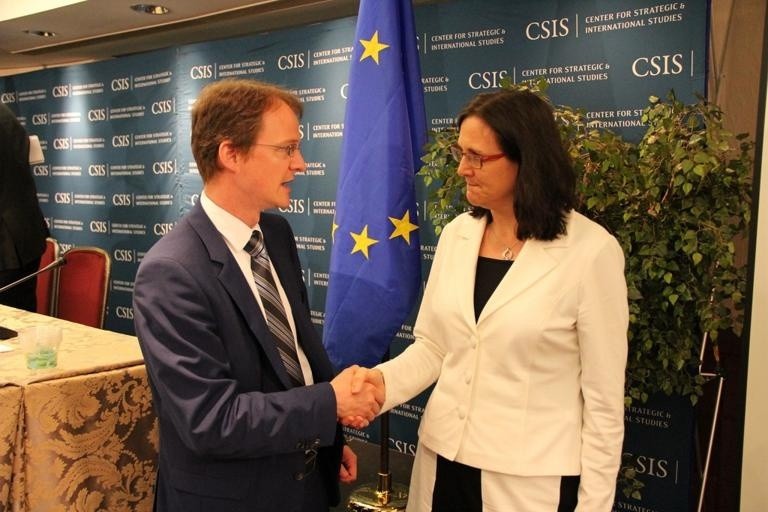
[0,257,67,293]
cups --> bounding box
[19,327,63,369]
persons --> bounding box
[0,99,51,312]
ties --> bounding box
[243,230,305,387]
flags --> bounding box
[322,0,429,378]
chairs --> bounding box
[36,234,111,329]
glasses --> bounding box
[450,144,506,169]
[231,142,300,157]
